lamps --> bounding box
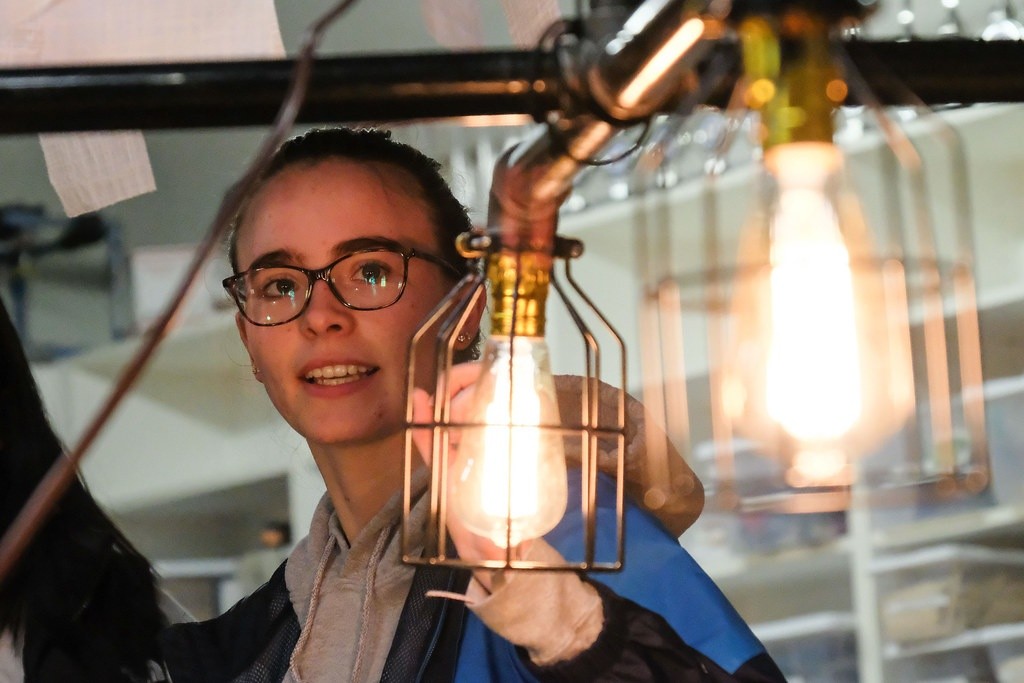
[629,18,999,517]
[398,0,709,571]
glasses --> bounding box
[222,248,463,327]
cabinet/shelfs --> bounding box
[38,110,1024,683]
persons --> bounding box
[168,126,787,683]
[2,299,176,682]
